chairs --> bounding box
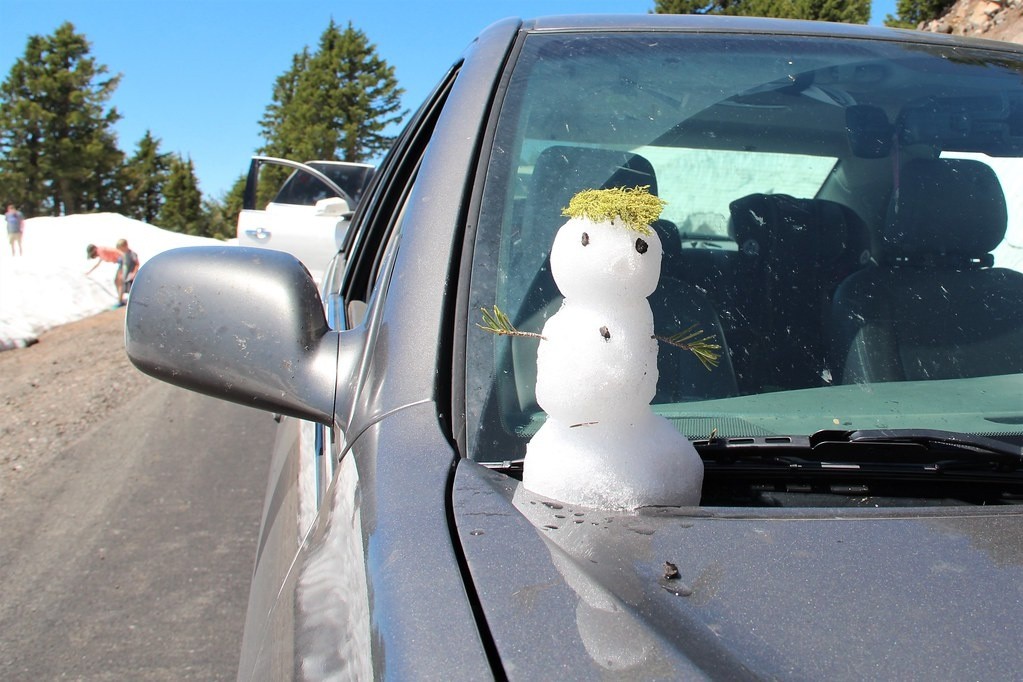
[832,158,1023,386]
[509,145,741,424]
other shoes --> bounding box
[112,302,124,309]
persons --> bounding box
[4,203,24,258]
[85,238,140,310]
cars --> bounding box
[236,155,378,301]
[122,11,1023,682]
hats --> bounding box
[87,244,96,256]
